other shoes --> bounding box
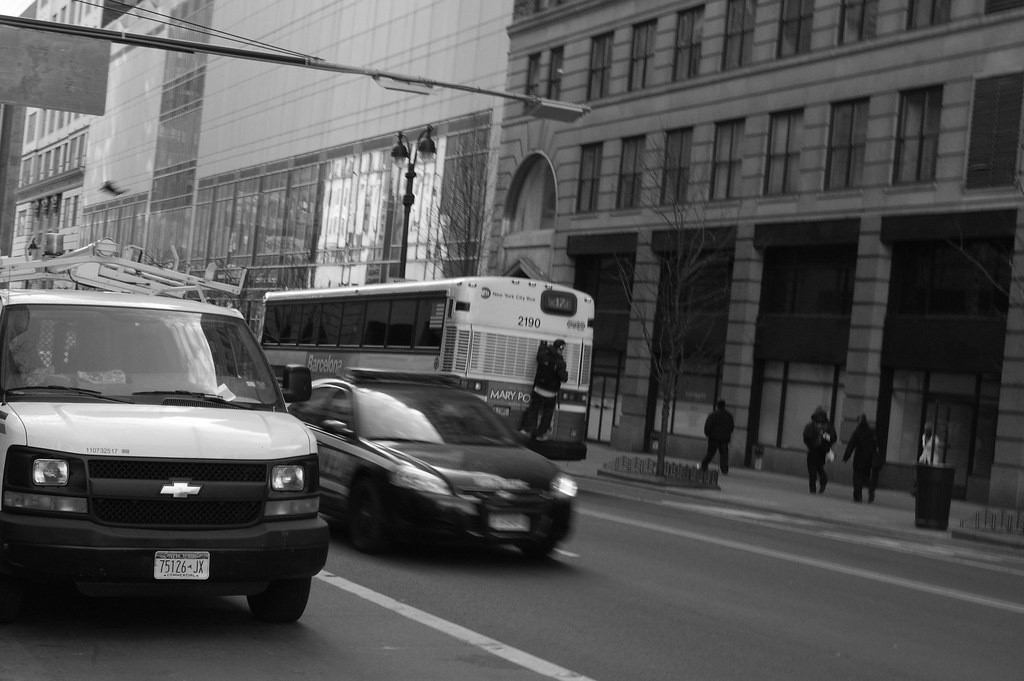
[520,430,531,438]
[536,435,548,441]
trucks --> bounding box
[0,235,331,626]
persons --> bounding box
[519,339,570,442]
[802,405,837,495]
[911,423,940,496]
[699,398,734,475]
[841,412,879,503]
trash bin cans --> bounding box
[751,443,765,472]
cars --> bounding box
[280,375,578,554]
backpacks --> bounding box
[540,347,558,384]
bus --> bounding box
[249,275,597,461]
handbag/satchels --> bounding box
[825,448,834,463]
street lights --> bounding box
[388,122,438,276]
